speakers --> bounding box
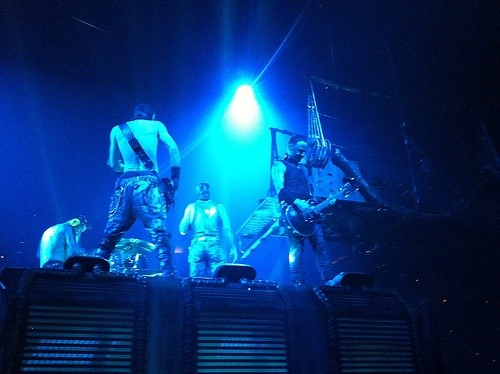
[63,255,110,273]
[327,272,374,288]
[214,264,256,283]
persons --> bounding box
[272,134,336,287]
[178,182,237,276]
[40,215,88,269]
[94,103,181,277]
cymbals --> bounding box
[115,238,157,255]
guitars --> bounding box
[106,158,176,213]
[280,180,356,243]
